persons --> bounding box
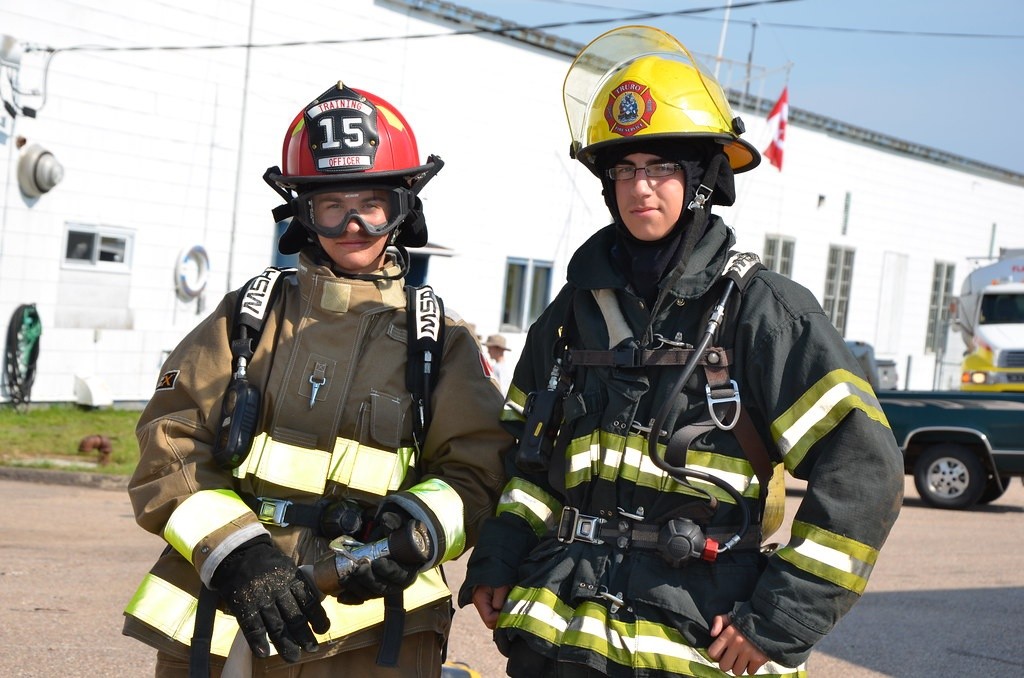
[120,79,506,678]
[457,24,905,678]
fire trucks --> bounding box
[944,248,1024,394]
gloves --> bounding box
[334,503,430,605]
[212,534,329,664]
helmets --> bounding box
[280,80,441,192]
[570,55,762,173]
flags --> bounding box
[763,86,788,173]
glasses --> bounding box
[608,159,684,181]
[287,186,416,239]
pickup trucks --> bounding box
[845,339,1024,511]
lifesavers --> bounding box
[174,242,211,300]
